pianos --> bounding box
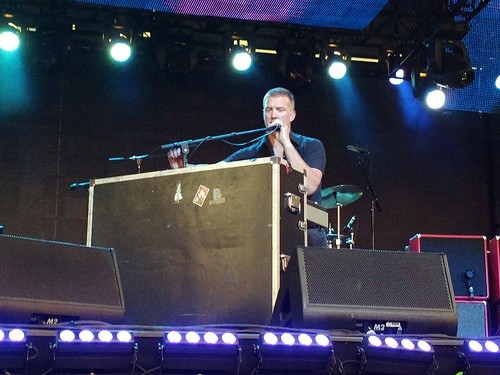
[85,156,329,326]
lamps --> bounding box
[0,13,475,109]
[0,328,500,375]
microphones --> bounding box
[276,123,281,129]
[343,215,355,233]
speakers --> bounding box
[0,234,124,325]
[271,234,500,339]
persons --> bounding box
[168,88,327,248]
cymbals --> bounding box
[321,184,363,208]
